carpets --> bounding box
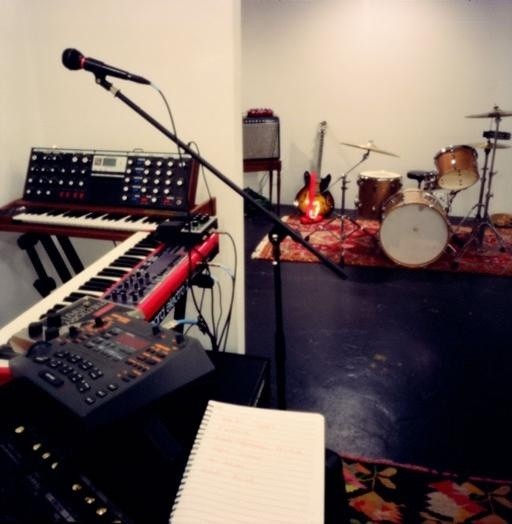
[337,448,512,524]
[251,214,512,275]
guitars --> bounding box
[294,121,334,220]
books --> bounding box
[168,400,325,524]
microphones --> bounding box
[62,48,152,86]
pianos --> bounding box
[0,231,219,386]
[0,197,217,232]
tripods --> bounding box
[304,175,377,268]
[449,119,508,270]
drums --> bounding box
[434,145,479,190]
[378,188,452,268]
[355,171,403,229]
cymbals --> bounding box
[470,139,512,151]
[340,141,400,159]
[464,110,512,119]
